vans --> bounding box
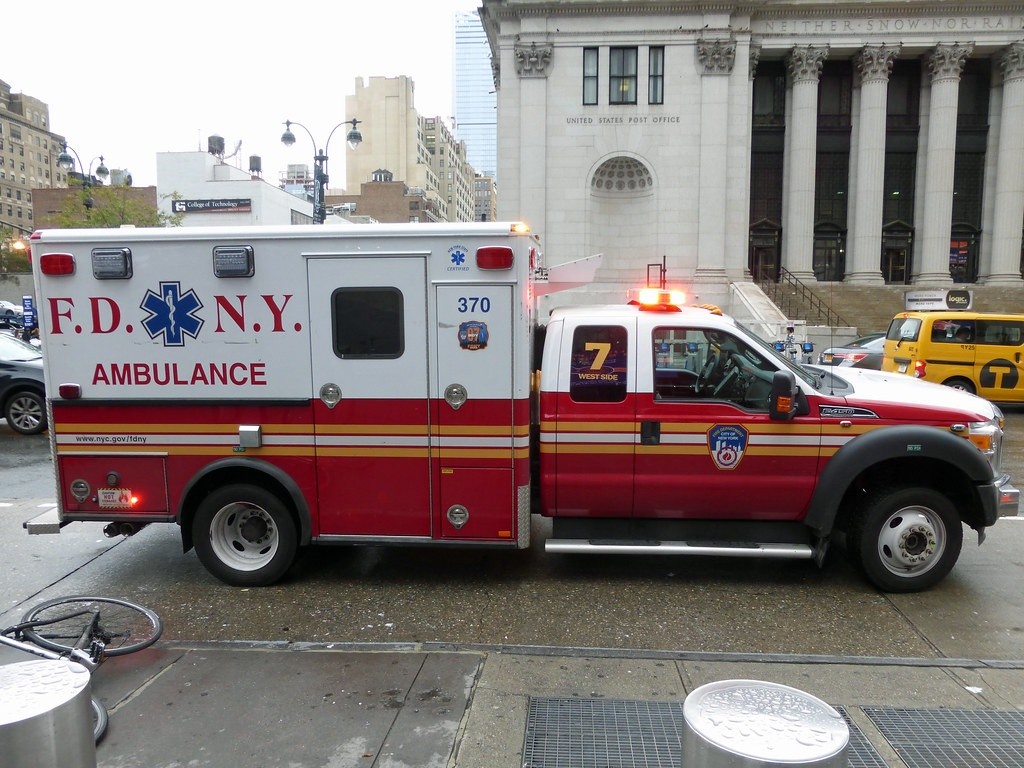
[880,308,1024,404]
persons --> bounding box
[952,327,970,339]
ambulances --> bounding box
[22,219,1020,595]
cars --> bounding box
[0,301,24,317]
[0,332,48,434]
[815,331,886,371]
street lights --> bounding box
[280,118,364,222]
[56,142,110,220]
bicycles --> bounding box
[0,594,164,747]
[4,320,39,342]
